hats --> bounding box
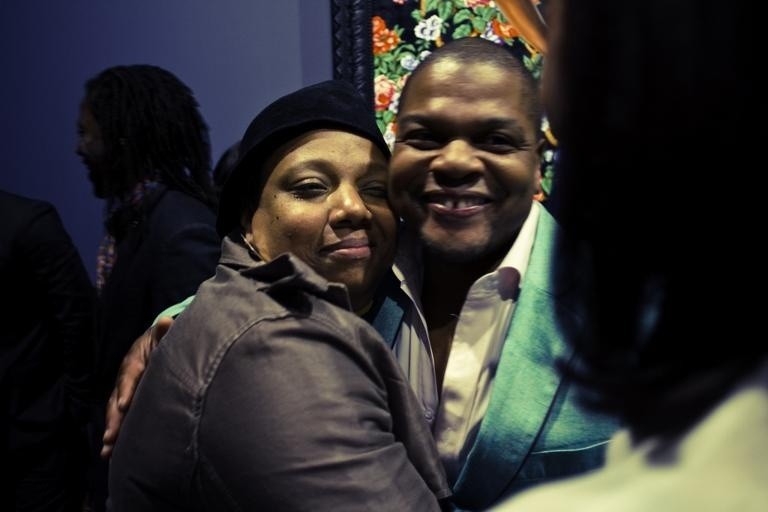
[213,80,393,237]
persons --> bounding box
[102,76,458,510]
[2,190,114,506]
[484,0,768,508]
[95,35,634,511]
[76,62,225,367]
